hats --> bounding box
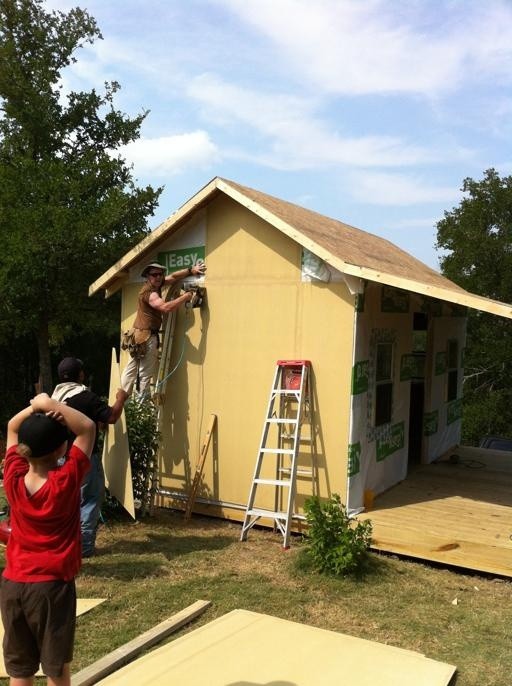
[141,262,168,278]
[57,357,84,378]
[18,412,69,458]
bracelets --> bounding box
[189,268,192,276]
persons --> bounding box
[121,261,207,397]
[51,357,129,558]
[1,392,96,686]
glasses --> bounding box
[148,272,163,277]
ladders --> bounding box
[139,296,177,517]
[240,359,316,549]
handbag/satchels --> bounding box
[121,328,152,360]
